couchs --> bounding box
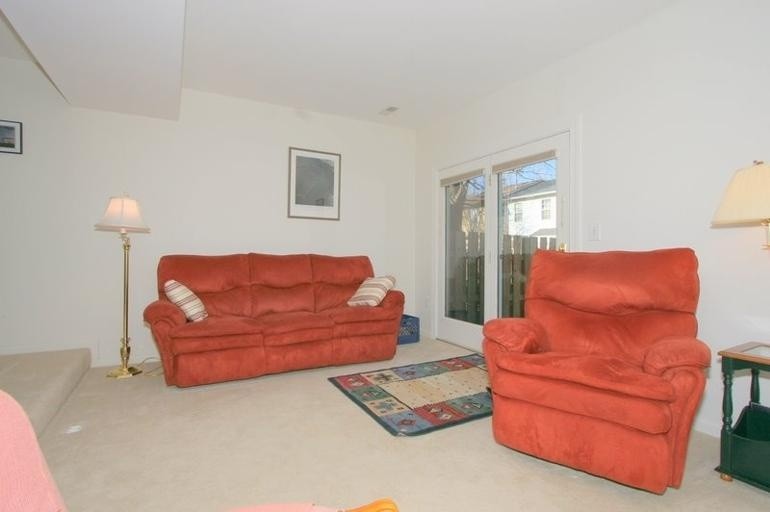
[142,253,404,388]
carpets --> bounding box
[327,349,494,438]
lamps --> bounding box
[92,193,151,379]
[712,160,770,248]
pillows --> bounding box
[163,276,208,323]
[346,276,396,307]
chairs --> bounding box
[0,388,400,512]
[483,248,712,496]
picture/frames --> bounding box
[0,119,23,155]
[288,146,340,221]
